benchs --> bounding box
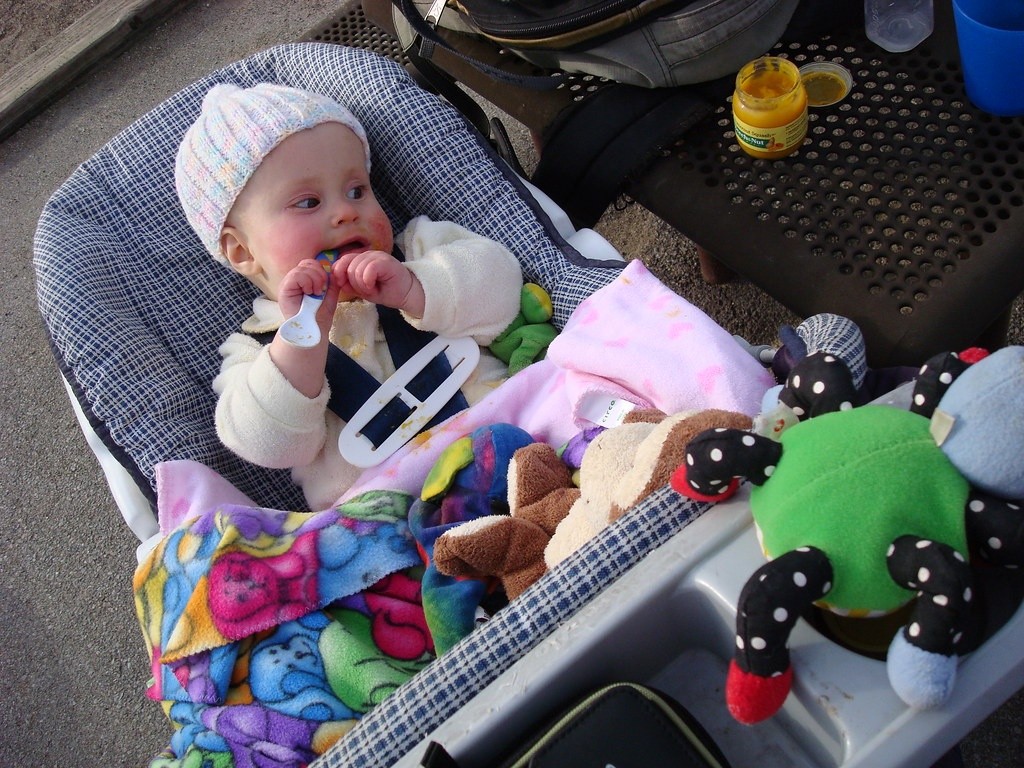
[362,0,1024,368]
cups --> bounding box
[865,0,934,54]
[952,0,1024,117]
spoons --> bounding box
[279,248,337,349]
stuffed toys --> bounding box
[486,282,558,377]
[671,346,1024,728]
[432,402,753,608]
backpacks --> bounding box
[391,0,799,231]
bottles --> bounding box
[733,57,810,159]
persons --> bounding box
[172,81,525,513]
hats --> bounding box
[175,83,371,269]
